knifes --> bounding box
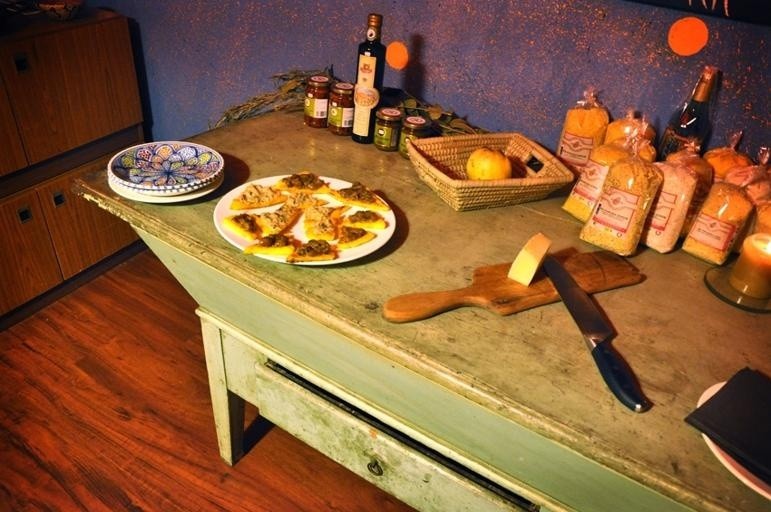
[541,248,650,412]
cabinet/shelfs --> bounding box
[0,14,151,192]
[2,141,145,318]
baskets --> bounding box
[406,132,575,212]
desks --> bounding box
[67,98,770,512]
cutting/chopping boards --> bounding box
[381,248,644,324]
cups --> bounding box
[729,231,771,300]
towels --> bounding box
[683,358,770,486]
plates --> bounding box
[696,380,771,504]
[107,140,225,203]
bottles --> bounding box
[350,14,386,145]
[656,64,720,161]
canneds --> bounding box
[305,76,426,160]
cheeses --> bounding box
[507,233,551,287]
